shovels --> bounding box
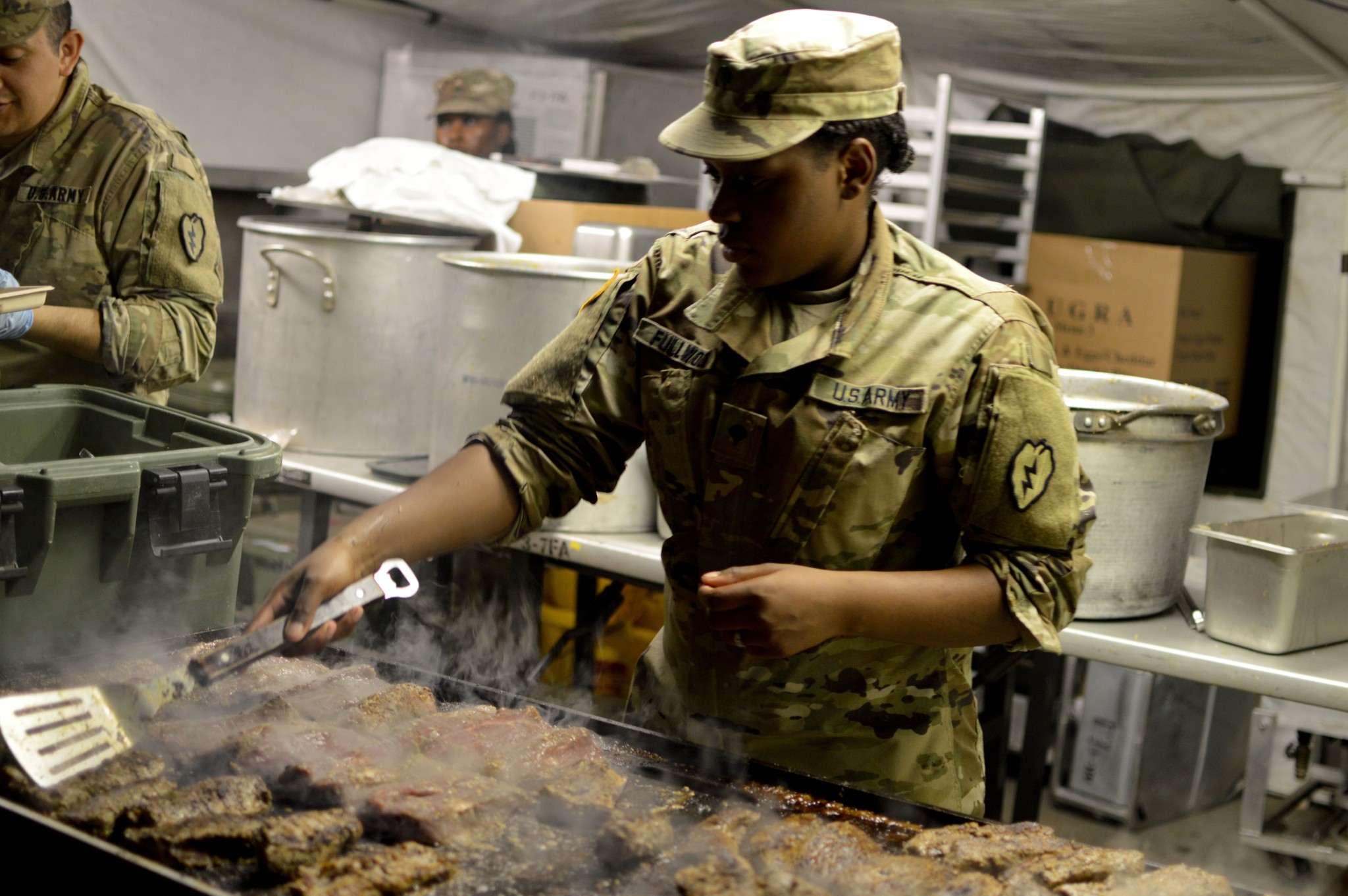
[0,556,423,793]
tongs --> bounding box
[1173,578,1208,635]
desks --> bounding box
[204,412,1348,896]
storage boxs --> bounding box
[1026,232,1248,436]
[1188,509,1347,656]
[0,383,284,669]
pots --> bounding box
[234,214,484,457]
[1059,365,1230,617]
[428,248,660,534]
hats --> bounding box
[0,0,67,47]
[656,7,904,162]
[426,70,517,122]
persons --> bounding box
[247,10,1097,826]
[425,69,516,157]
[0,1,224,406]
